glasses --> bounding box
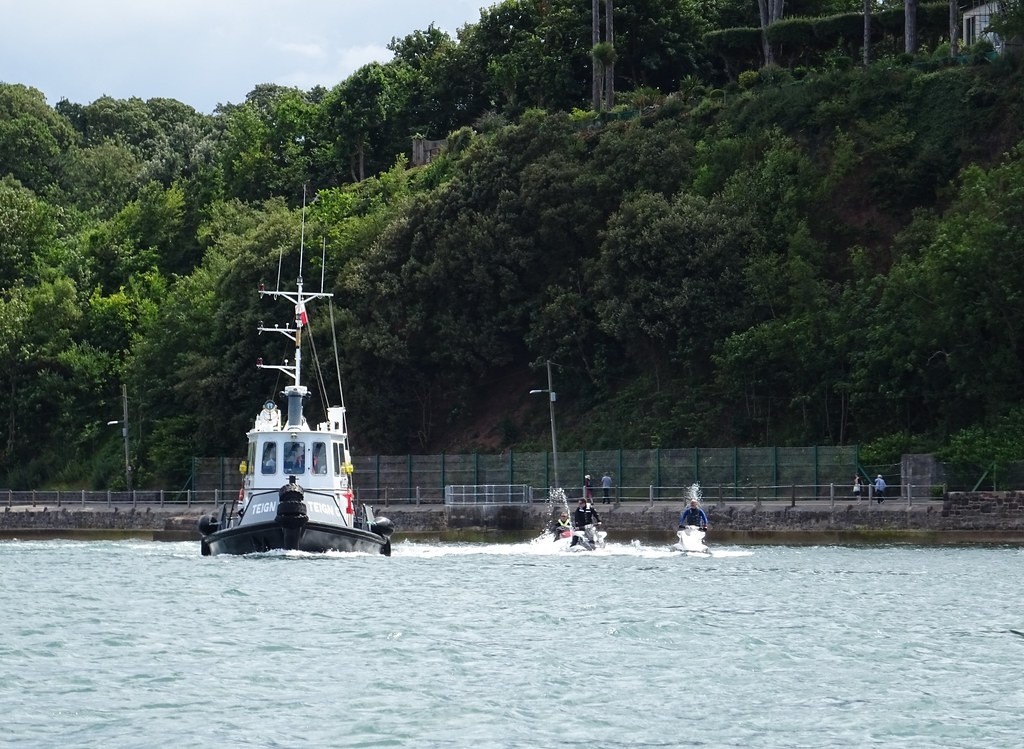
[561,516,566,517]
[579,502,584,505]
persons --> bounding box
[601,472,613,505]
[678,500,708,529]
[571,498,602,546]
[554,512,575,539]
[875,474,886,504]
[296,450,317,473]
[584,475,594,505]
[853,473,864,505]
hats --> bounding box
[585,475,590,480]
[878,475,882,478]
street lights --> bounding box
[528,362,559,491]
[107,383,131,491]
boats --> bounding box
[197,183,396,557]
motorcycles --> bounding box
[571,523,606,551]
[676,525,709,553]
[553,527,572,543]
